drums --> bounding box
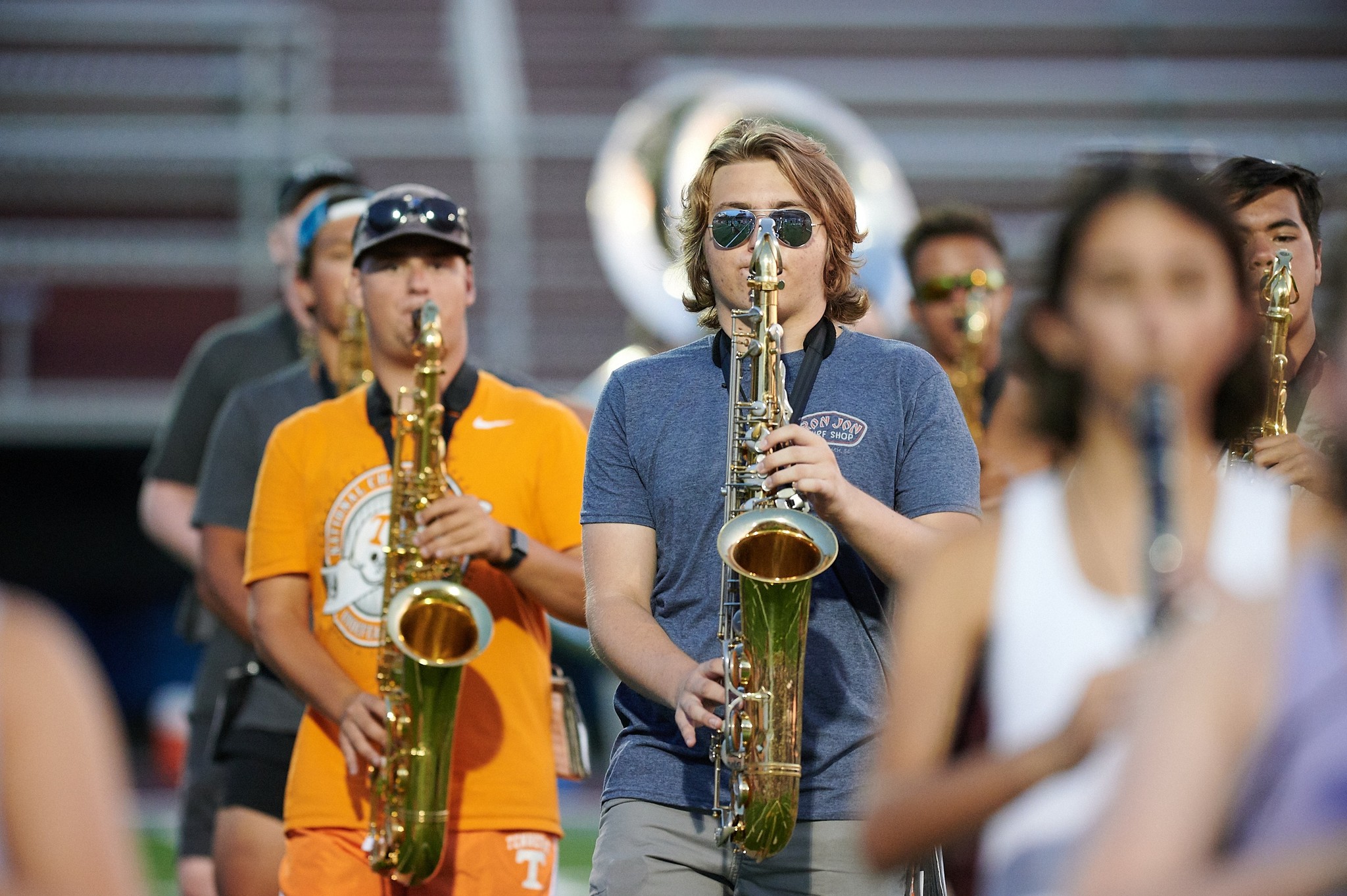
[546,661,594,791]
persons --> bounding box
[862,149,1347,896]
[580,118,977,896]
[137,152,590,896]
[899,206,1045,896]
[1195,147,1347,504]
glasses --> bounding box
[707,208,824,250]
[350,196,471,244]
[916,267,1011,303]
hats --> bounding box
[276,164,361,215]
[296,185,378,273]
[349,182,474,266]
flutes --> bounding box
[1128,367,1190,643]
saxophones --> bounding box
[944,279,997,444]
[339,264,373,397]
[709,218,841,867]
[1224,248,1301,507]
[358,295,494,886]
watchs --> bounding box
[487,524,529,574]
